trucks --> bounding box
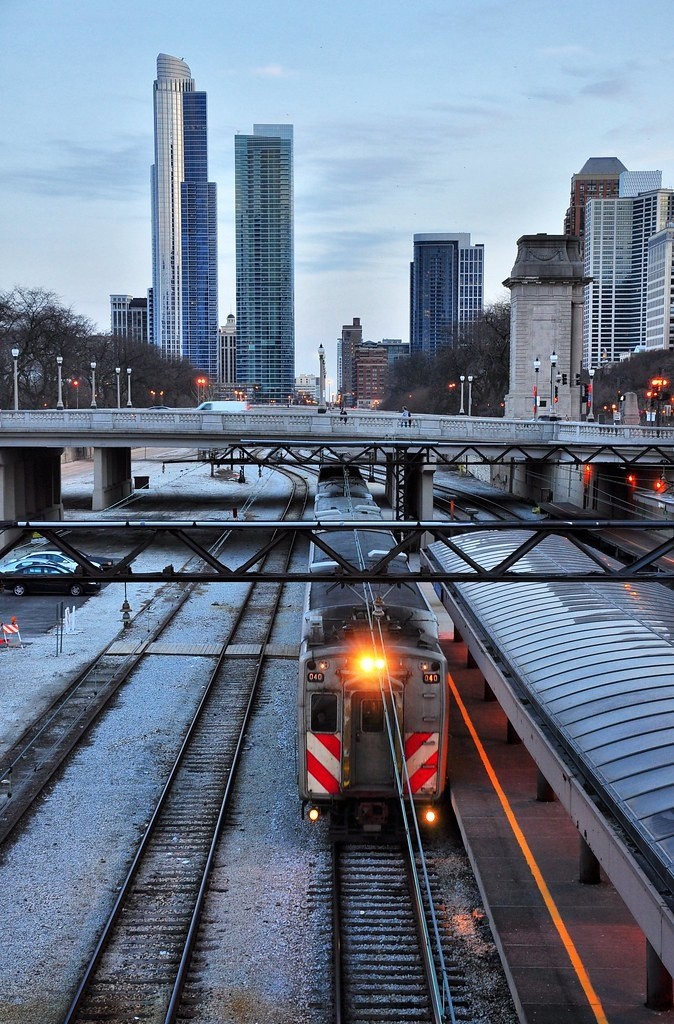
[195,401,246,411]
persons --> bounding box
[340,406,347,425]
[402,406,412,427]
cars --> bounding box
[0,564,101,596]
[149,406,169,410]
[0,558,74,574]
[4,551,103,570]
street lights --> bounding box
[91,361,97,409]
[115,365,132,408]
[57,353,64,410]
[588,367,595,423]
[460,373,466,415]
[647,379,667,438]
[468,373,473,418]
[318,343,334,413]
[534,351,558,421]
[198,379,205,406]
[74,381,78,408]
[12,347,19,410]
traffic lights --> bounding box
[562,373,580,385]
[618,394,626,402]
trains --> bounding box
[298,466,449,844]
